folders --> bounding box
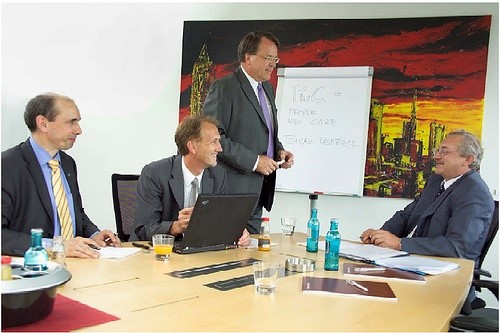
[340,244,461,276]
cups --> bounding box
[152,234,174,261]
[252,260,280,295]
[281,217,297,237]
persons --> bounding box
[129,116,251,246]
[203,31,294,233]
[1,93,122,259]
[361,129,495,303]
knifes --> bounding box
[354,267,386,271]
[346,278,368,292]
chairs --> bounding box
[461,200,499,313]
[112,174,140,242]
[450,280,499,332]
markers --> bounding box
[277,158,288,165]
[314,191,358,196]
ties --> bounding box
[257,83,275,159]
[48,159,74,240]
[188,178,199,208]
[431,182,446,203]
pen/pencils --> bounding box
[355,267,386,271]
[346,278,368,292]
[132,243,149,249]
[358,236,371,240]
[104,233,117,243]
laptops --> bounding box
[148,193,259,254]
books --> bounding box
[241,237,278,249]
[91,246,141,259]
[297,239,459,302]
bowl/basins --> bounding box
[1,261,72,329]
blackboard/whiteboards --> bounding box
[273,66,374,196]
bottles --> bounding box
[258,217,270,252]
[1,256,12,281]
[42,236,65,288]
[306,208,320,253]
[24,228,49,260]
[324,220,341,271]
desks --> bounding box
[1,232,473,333]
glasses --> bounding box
[252,53,280,63]
[435,149,456,156]
